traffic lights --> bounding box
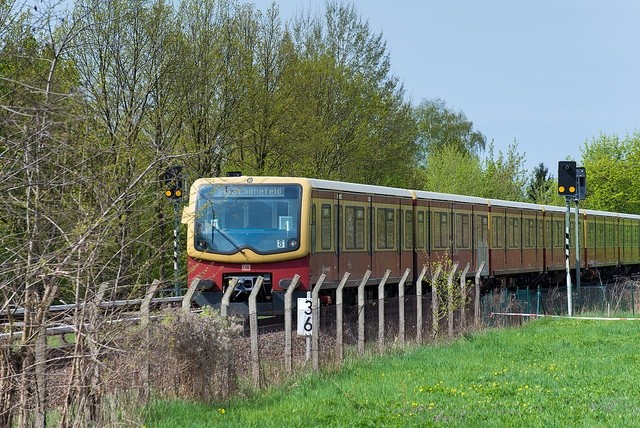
[166,167,182,200]
[558,162,576,195]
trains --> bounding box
[181,172,640,316]
